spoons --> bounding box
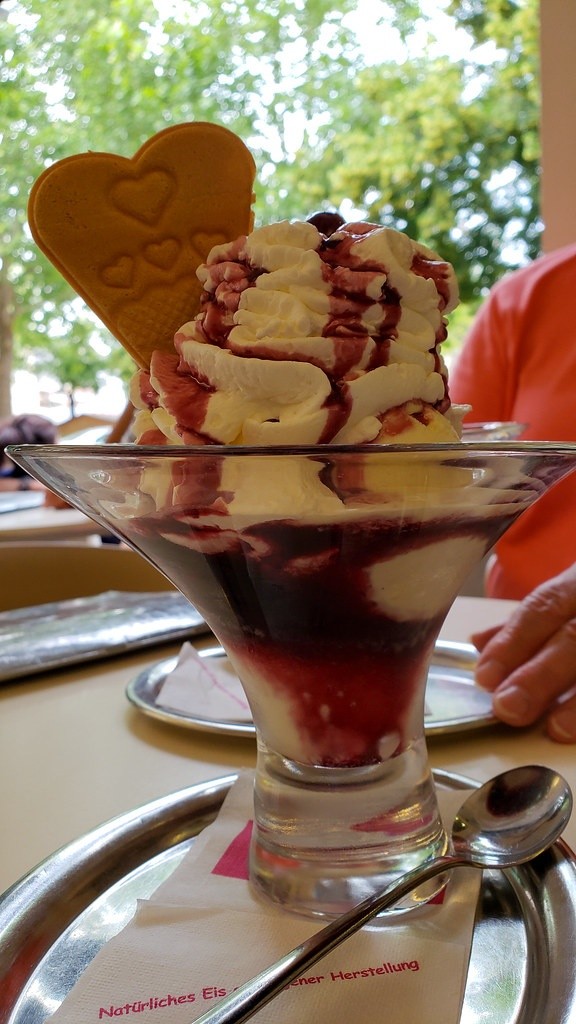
[192,763,574,1024]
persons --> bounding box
[447,243,576,747]
[0,416,58,491]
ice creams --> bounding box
[122,210,544,768]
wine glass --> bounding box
[4,442,576,922]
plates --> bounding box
[125,640,502,738]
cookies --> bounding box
[28,121,259,373]
